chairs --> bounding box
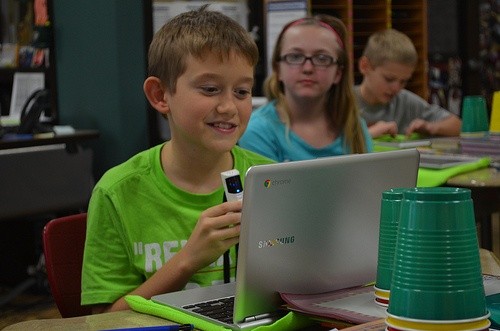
[43,212,89,318]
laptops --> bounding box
[152,148,420,331]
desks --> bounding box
[0,129,500,331]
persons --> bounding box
[81,11,279,315]
[354,29,461,138]
[234,16,375,164]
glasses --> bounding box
[278,53,338,66]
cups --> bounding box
[373,188,493,331]
[459,91,500,138]
